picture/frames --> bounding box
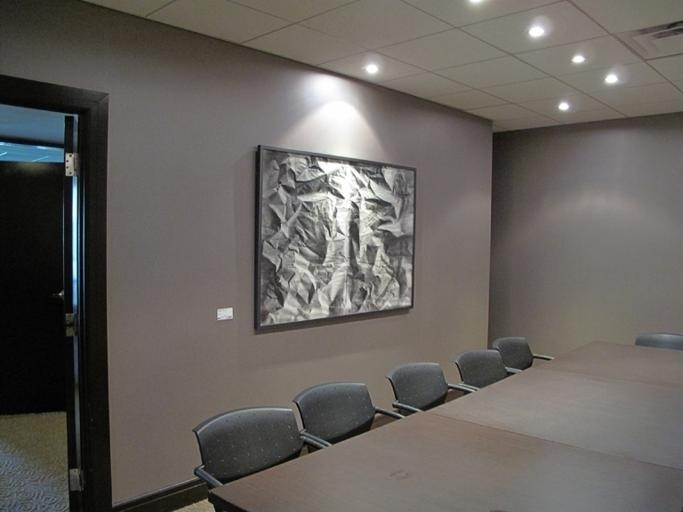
[253,144,416,334]
